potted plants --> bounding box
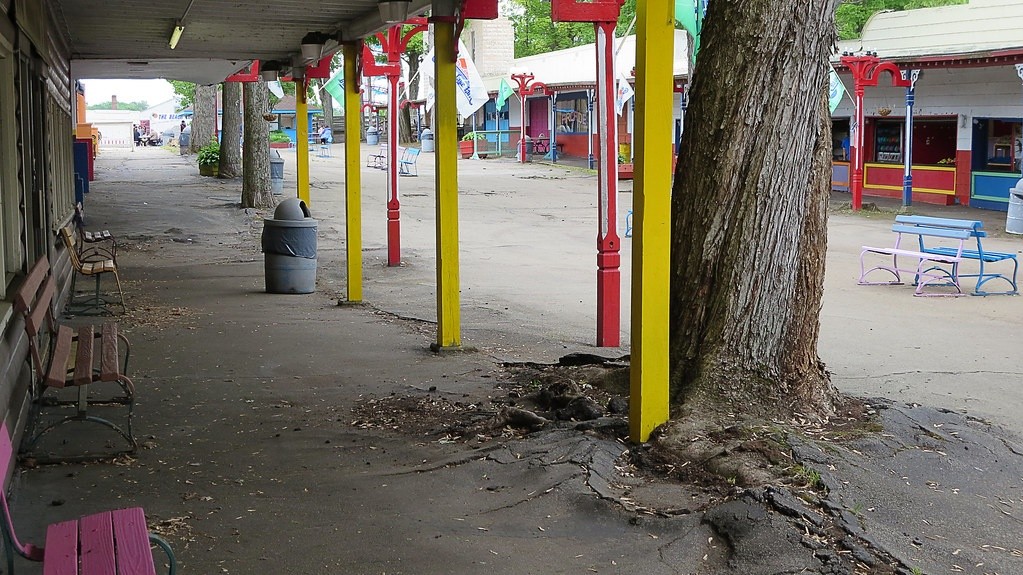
[459,132,489,159]
[197,135,220,177]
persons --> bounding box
[317,124,334,145]
[132,124,146,146]
[180,120,186,134]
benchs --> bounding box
[857,215,975,297]
[366,144,387,172]
[398,147,420,177]
[910,215,1021,296]
[11,255,136,468]
[59,227,126,316]
[625,210,632,239]
[308,137,336,158]
[0,422,176,575]
[134,138,164,148]
[74,202,118,269]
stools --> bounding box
[288,142,294,152]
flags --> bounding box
[616,75,635,118]
[324,71,345,109]
[827,64,845,114]
[267,76,285,99]
[495,77,513,111]
[418,37,490,118]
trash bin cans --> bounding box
[179,124,191,154]
[420,128,434,152]
[1005,179,1023,235]
[365,126,379,145]
[263,197,318,295]
[270,147,284,195]
[517,135,533,162]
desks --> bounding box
[138,136,152,146]
[531,136,551,140]
[307,133,322,139]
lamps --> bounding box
[300,31,342,61]
[168,19,185,50]
[377,0,412,23]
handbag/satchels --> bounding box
[327,135,333,143]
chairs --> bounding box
[530,133,564,155]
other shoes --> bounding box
[322,142,325,144]
